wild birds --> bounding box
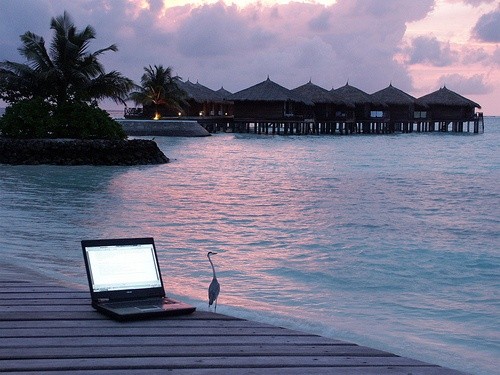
[207,251,222,313]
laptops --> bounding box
[80,236,197,323]
